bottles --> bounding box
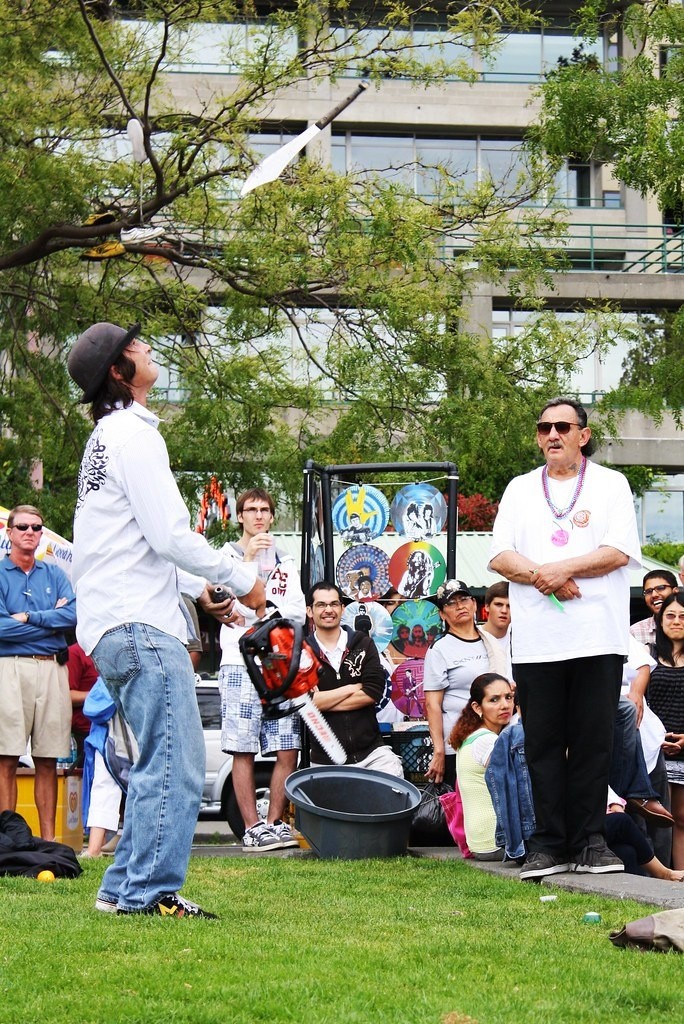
[213,587,232,615]
[56,734,78,769]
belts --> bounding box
[14,654,55,660]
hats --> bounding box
[437,579,473,612]
[67,321,142,403]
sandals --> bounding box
[628,798,675,828]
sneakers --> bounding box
[268,819,300,848]
[570,834,625,873]
[518,851,571,878]
[117,892,219,919]
[95,899,118,912]
[240,821,283,852]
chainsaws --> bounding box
[239,599,348,767]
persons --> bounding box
[393,625,437,657]
[355,605,372,637]
[485,397,643,880]
[402,503,435,537]
[423,579,501,783]
[394,670,423,716]
[353,576,377,602]
[398,551,434,597]
[0,504,77,843]
[295,582,406,780]
[66,320,267,922]
[342,513,372,542]
[451,555,684,882]
[218,487,308,853]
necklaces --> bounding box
[541,456,587,547]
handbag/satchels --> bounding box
[410,782,455,847]
[437,779,474,859]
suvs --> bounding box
[187,676,301,843]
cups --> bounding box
[259,535,275,571]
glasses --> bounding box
[536,422,580,435]
[310,602,342,608]
[242,507,271,514]
[12,523,43,531]
[443,596,472,608]
[642,585,671,596]
